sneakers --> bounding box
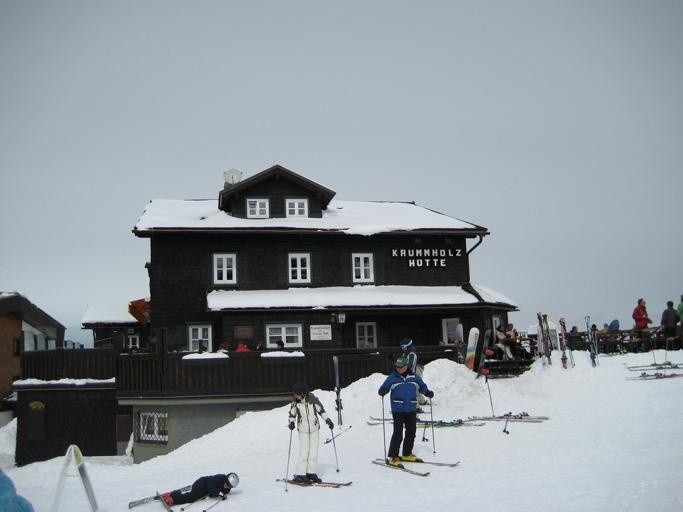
[292,472,323,485]
[401,453,425,463]
[385,456,405,470]
[163,492,174,507]
[416,406,424,414]
[418,399,429,406]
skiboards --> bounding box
[584,316,597,368]
[276,474,350,489]
[370,455,459,477]
[537,312,554,366]
[624,361,683,381]
[128,485,191,511]
[364,414,550,429]
[334,356,344,427]
[560,318,568,368]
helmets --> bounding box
[399,337,414,348]
[225,471,240,489]
[393,357,408,367]
[291,381,308,395]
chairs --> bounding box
[557,322,683,354]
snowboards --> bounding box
[455,324,465,365]
[476,328,494,377]
[465,327,478,368]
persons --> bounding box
[399,337,425,421]
[661,301,680,350]
[437,324,533,363]
[378,356,433,468]
[199,339,285,352]
[632,298,652,352]
[163,473,240,507]
[676,295,683,349]
[568,324,617,354]
[288,382,334,483]
